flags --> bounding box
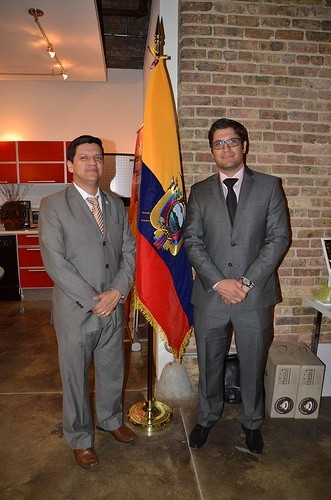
[128,56,194,361]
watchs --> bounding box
[242,276,254,290]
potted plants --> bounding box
[0,201,27,231]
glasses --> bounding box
[211,138,243,150]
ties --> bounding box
[223,177,239,227]
[86,196,104,236]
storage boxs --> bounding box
[262,341,326,420]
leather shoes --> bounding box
[96,421,137,443]
[240,423,265,454]
[73,446,100,470]
[188,422,214,448]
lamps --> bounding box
[27,8,69,81]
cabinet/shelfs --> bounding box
[0,140,72,184]
[17,231,56,290]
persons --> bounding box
[38,136,137,470]
[183,119,290,453]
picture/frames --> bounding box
[29,209,39,227]
